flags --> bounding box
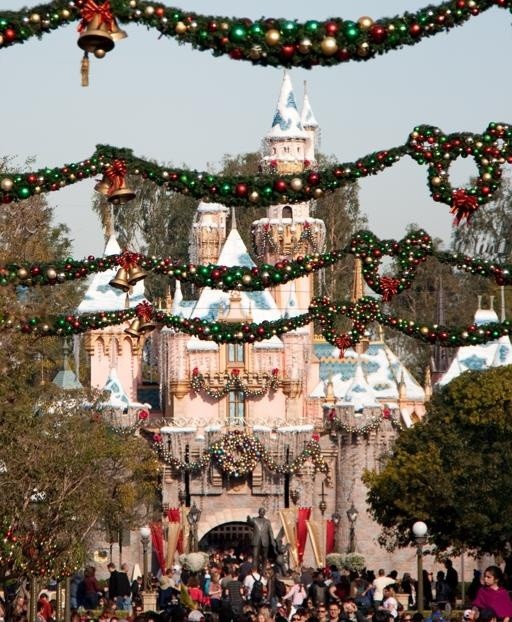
[168,505,191,552]
[305,516,336,566]
[278,505,312,565]
[151,522,180,573]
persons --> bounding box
[246,508,276,573]
[274,541,288,575]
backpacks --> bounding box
[250,573,265,602]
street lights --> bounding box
[140,522,151,589]
[412,521,427,612]
[346,501,358,552]
[186,501,202,552]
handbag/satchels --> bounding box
[397,602,405,612]
